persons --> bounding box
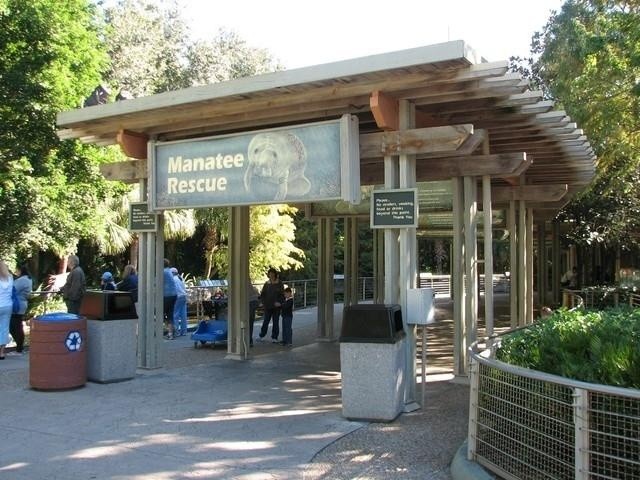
[101,257,188,340]
[6,261,33,356]
[248,276,259,347]
[254,267,284,343]
[277,288,294,349]
[560,262,613,309]
[57,254,89,315]
[0,262,14,360]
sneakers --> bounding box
[271,338,279,343]
[279,341,286,347]
[287,343,293,349]
[7,351,23,356]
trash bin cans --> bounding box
[29,313,87,389]
[339,304,407,423]
[79,289,139,383]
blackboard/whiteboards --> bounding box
[370,188,418,228]
[128,203,158,233]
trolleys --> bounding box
[190,288,228,350]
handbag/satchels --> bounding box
[12,285,20,313]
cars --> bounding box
[493,273,507,291]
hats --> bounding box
[101,272,112,280]
[170,268,178,273]
